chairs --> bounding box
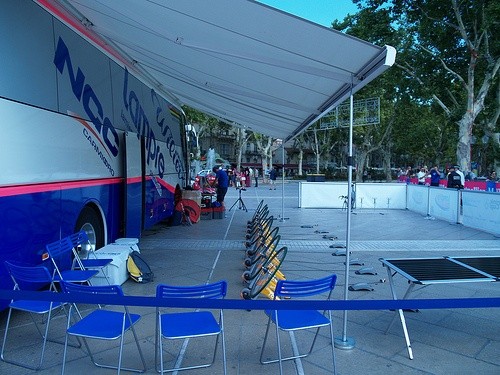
[259,273,337,375]
[155,279,228,375]
[0,261,82,375]
[60,280,147,375]
[41,229,113,325]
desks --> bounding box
[378,255,500,359]
[202,193,215,220]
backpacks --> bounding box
[127,246,154,283]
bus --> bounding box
[0,0,189,317]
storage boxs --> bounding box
[89,241,140,286]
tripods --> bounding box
[229,188,247,212]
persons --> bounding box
[268,168,277,191]
[418,167,428,186]
[210,165,228,206]
[252,167,259,187]
[228,166,251,190]
[429,166,441,186]
[469,168,478,181]
[446,166,466,206]
[396,165,421,178]
[485,171,499,183]
